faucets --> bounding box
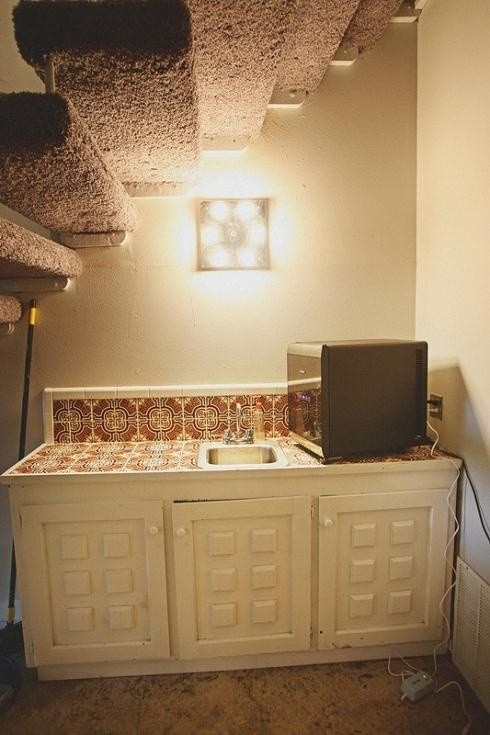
[235,402,242,437]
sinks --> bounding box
[197,442,289,468]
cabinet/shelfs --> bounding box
[314,488,448,648]
[16,494,314,665]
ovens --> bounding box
[284,339,429,464]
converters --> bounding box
[400,669,434,704]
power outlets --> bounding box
[429,392,442,420]
